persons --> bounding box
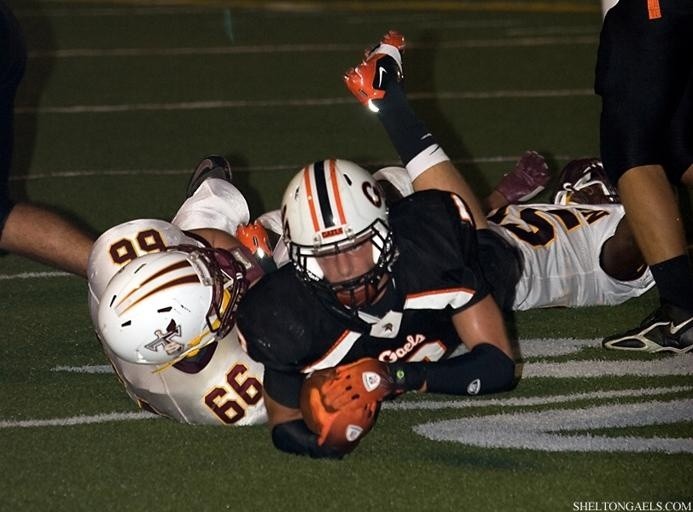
[0,0,693,459]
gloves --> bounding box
[319,397,380,453]
[318,358,394,409]
[494,148,553,204]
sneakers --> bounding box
[344,31,410,111]
[602,299,693,355]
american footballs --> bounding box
[301,367,380,446]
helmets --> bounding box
[98,246,249,365]
[552,157,622,203]
[281,158,400,321]
